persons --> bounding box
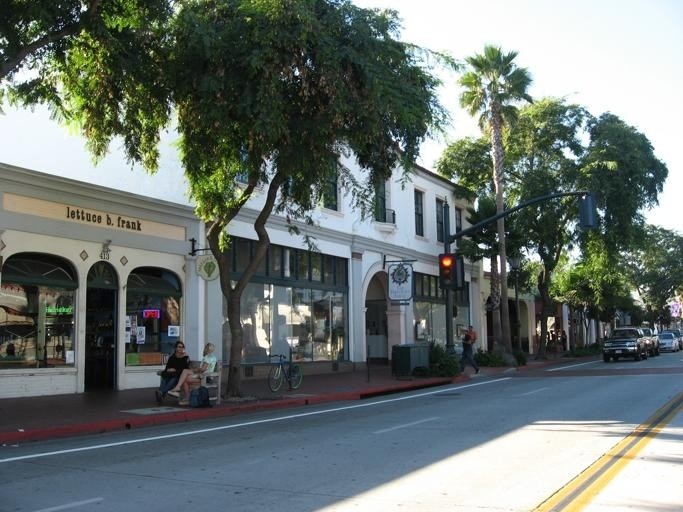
[545,329,567,351]
[154,341,191,404]
[166,342,217,406]
[466,325,478,346]
[458,327,479,374]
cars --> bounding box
[601,326,682,362]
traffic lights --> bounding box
[439,253,458,289]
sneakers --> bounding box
[155,390,163,401]
[168,389,181,398]
[179,399,189,405]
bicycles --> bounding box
[268,354,303,392]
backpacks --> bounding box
[189,386,208,408]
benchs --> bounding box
[156,360,223,407]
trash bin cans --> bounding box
[551,341,560,360]
